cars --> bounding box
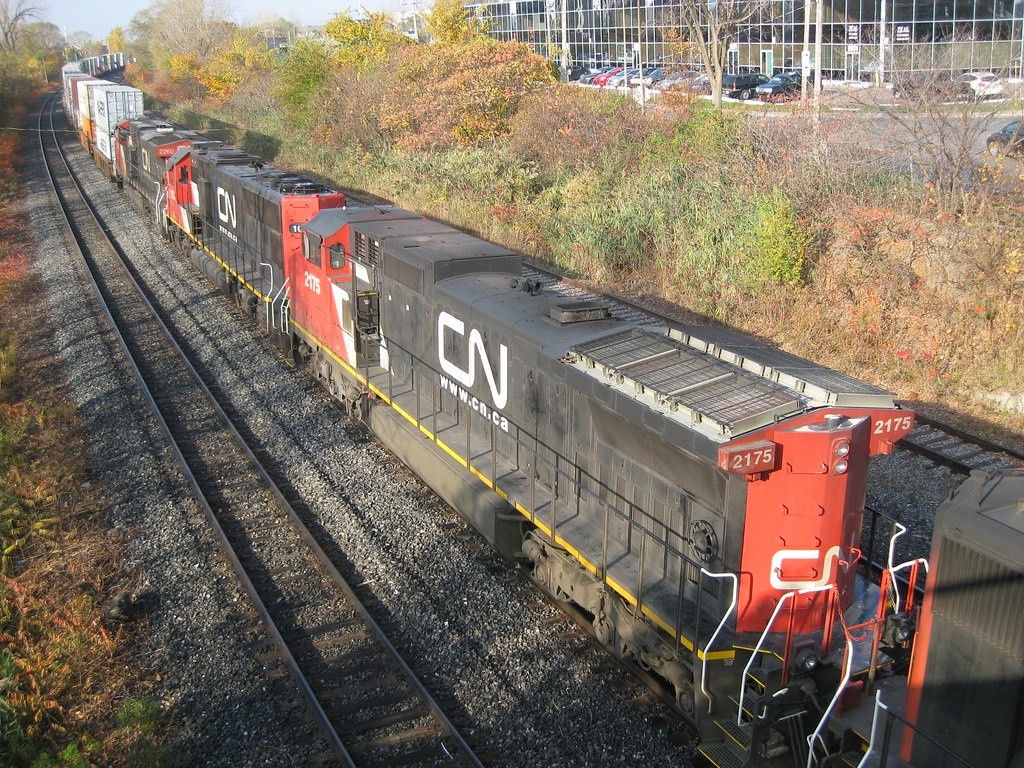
[952,71,1007,102]
[985,119,1024,159]
[891,71,954,102]
[569,65,592,82]
[580,65,716,95]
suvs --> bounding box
[755,69,823,103]
[722,73,771,101]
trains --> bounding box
[58,50,1024,768]
